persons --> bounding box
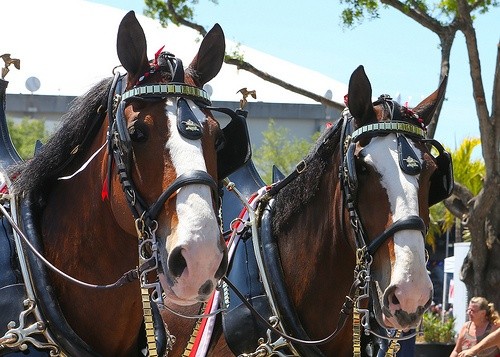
[450,297,500,357]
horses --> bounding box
[3,10,226,354]
[157,65,449,354]
